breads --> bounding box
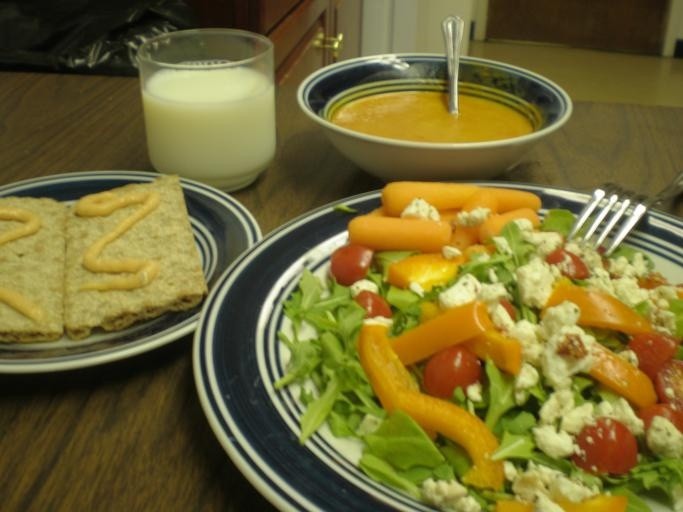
[63,173,210,338]
[0,197,63,342]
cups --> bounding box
[137,27,277,194]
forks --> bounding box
[566,169,683,256]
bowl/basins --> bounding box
[296,51,574,187]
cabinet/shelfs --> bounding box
[204,1,364,86]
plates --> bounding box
[191,187,683,511]
[1,170,263,374]
